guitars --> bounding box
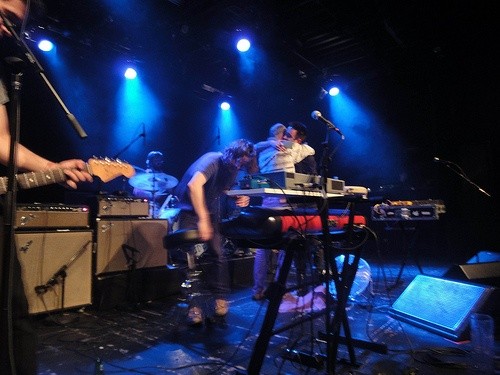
[0,155,136,195]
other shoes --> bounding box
[253,293,262,300]
[215,299,227,316]
[189,306,202,322]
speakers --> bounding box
[97,218,169,273]
[11,231,94,315]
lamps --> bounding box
[231,24,251,53]
[325,78,340,96]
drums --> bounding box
[135,199,160,219]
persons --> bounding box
[253,124,316,298]
[176,140,254,324]
[0,0,93,375]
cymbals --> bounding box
[128,172,178,192]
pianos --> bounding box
[217,171,370,375]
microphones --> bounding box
[433,156,453,166]
[123,244,140,254]
[35,269,64,294]
[311,110,343,136]
